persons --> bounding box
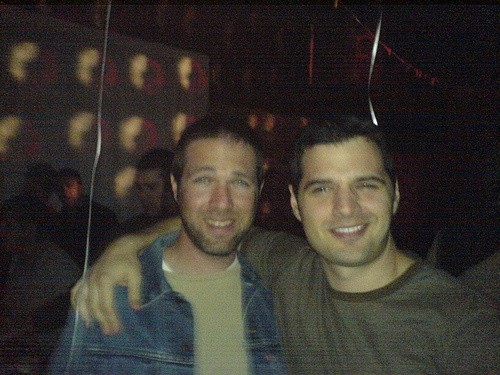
[129,148,180,231]
[71,115,500,375]
[0,163,120,375]
[48,113,288,375]
